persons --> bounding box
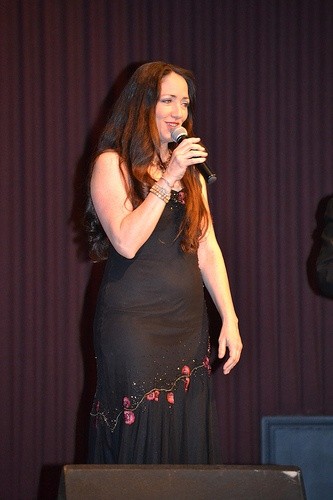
[83,60,245,466]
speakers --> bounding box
[58,463,307,499]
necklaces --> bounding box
[148,147,174,178]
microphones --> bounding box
[171,125,218,184]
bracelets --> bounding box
[161,177,174,189]
[148,182,172,203]
